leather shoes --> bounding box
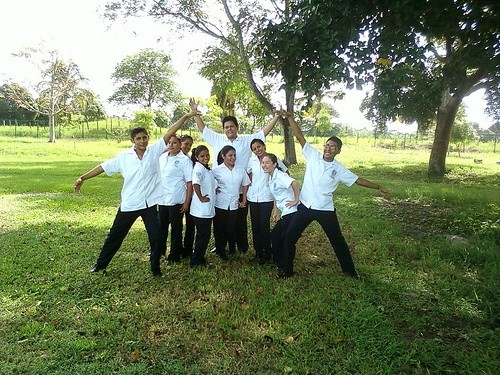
[259,259,267,265]
[210,247,216,253]
[191,262,213,268]
[219,255,229,262]
[229,253,235,256]
[276,271,294,280]
[347,269,361,280]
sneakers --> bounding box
[88,267,106,272]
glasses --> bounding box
[324,144,336,150]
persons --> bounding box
[178,134,196,259]
[189,97,282,253]
[261,153,300,265]
[211,145,252,262]
[240,138,290,261]
[189,144,216,268]
[275,108,391,281]
[157,135,193,265]
[74,110,203,277]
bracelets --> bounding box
[377,185,381,190]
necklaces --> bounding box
[229,136,238,145]
[135,149,146,164]
[224,162,234,171]
